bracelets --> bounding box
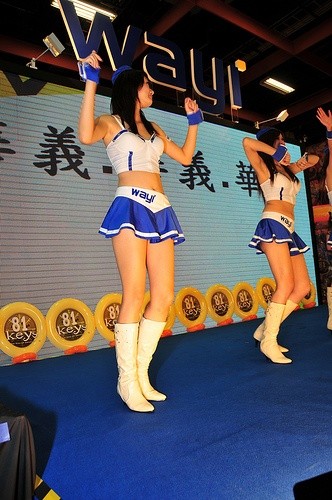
[77,62,101,84]
[305,152,308,161]
[186,109,204,125]
[271,144,288,163]
[327,131,332,140]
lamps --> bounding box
[49,0,122,25]
[25,32,66,71]
[234,57,247,73]
[255,108,290,129]
[258,75,296,95]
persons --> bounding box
[77,49,200,412]
[243,127,319,303]
[316,108,332,331]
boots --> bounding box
[136,314,166,401]
[114,322,154,412]
[326,287,332,329]
[259,301,292,363]
[252,300,298,353]
